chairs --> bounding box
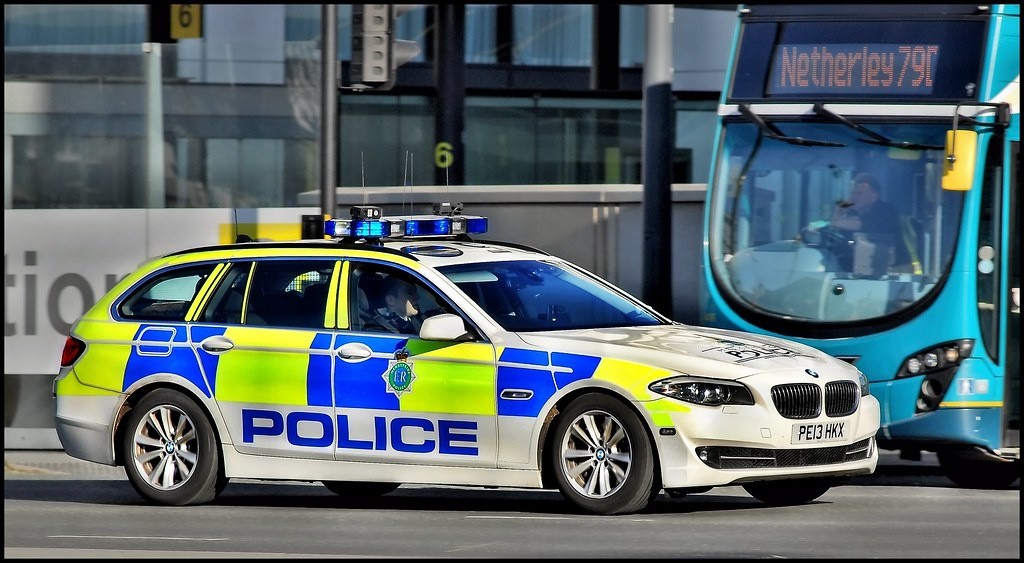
[360,274,385,327]
[226,280,327,326]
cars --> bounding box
[50,201,881,518]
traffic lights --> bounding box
[347,2,424,91]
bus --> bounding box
[698,1,1024,494]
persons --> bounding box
[833,175,911,266]
[362,274,420,334]
